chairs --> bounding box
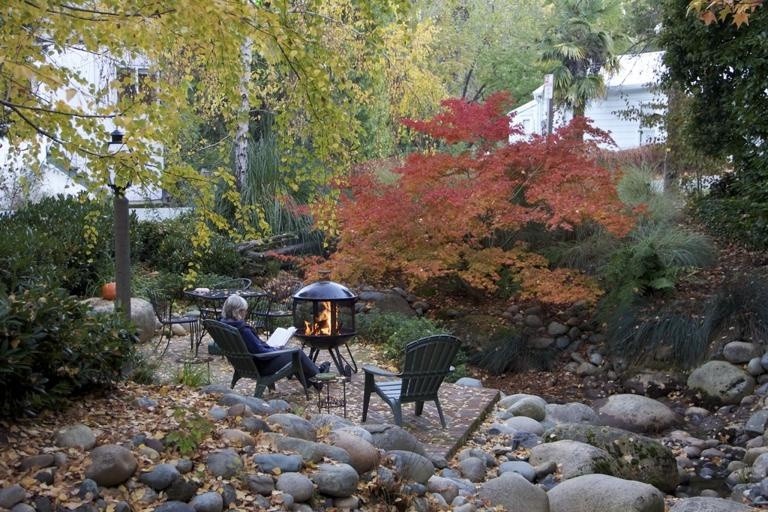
[146,277,311,399]
[361,333,463,430]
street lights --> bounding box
[103,130,137,323]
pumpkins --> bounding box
[103,283,116,299]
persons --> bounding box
[220,295,331,390]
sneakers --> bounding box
[314,361,330,390]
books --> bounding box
[266,326,299,349]
